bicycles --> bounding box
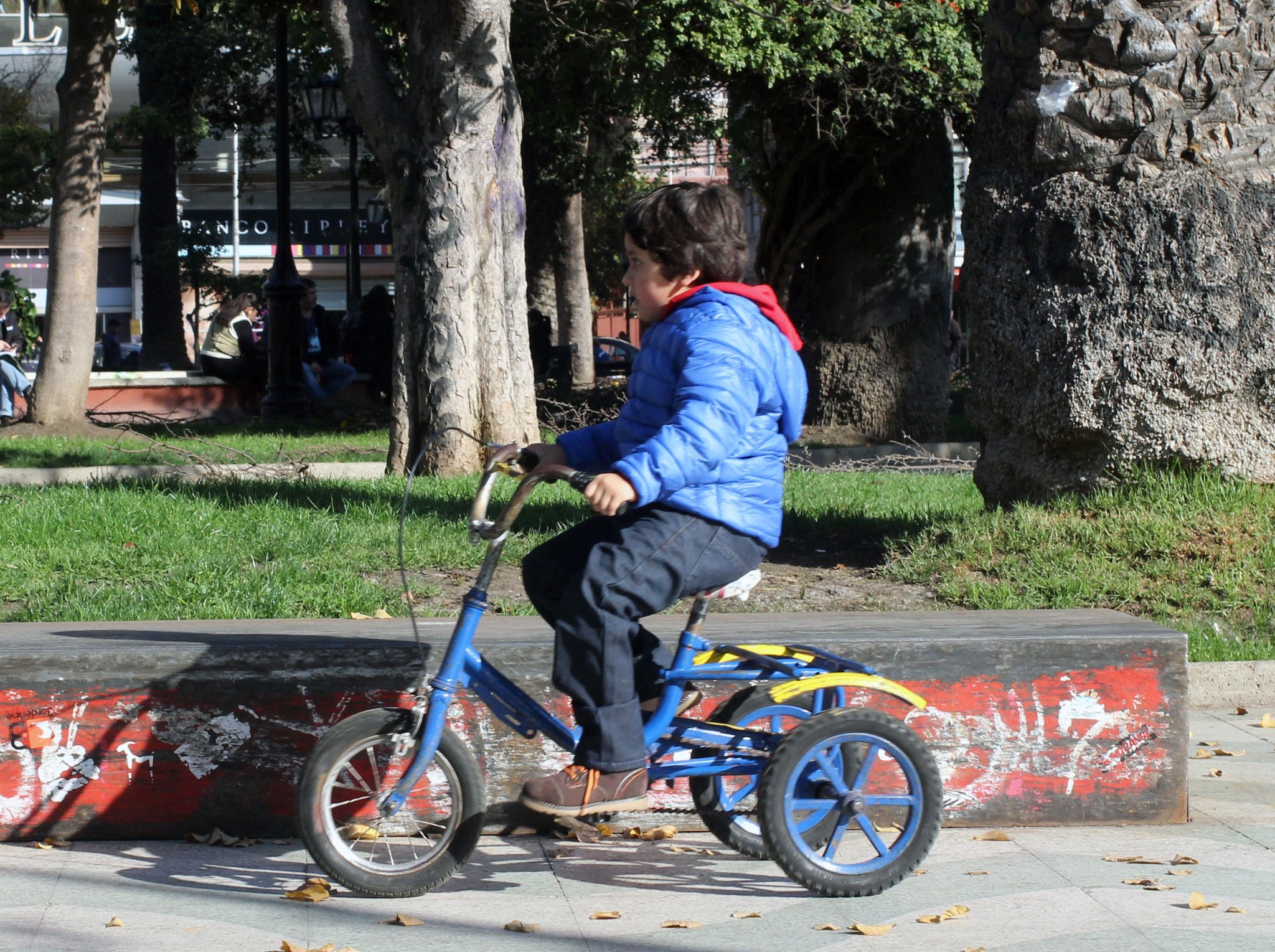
[298,425,944,899]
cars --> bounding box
[592,338,639,376]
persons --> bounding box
[337,282,403,399]
[294,275,356,405]
[198,273,269,413]
[611,331,631,359]
[0,287,37,426]
[98,316,128,371]
[593,341,611,363]
[526,307,560,375]
[477,181,809,821]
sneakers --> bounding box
[519,764,649,817]
[640,681,702,716]
